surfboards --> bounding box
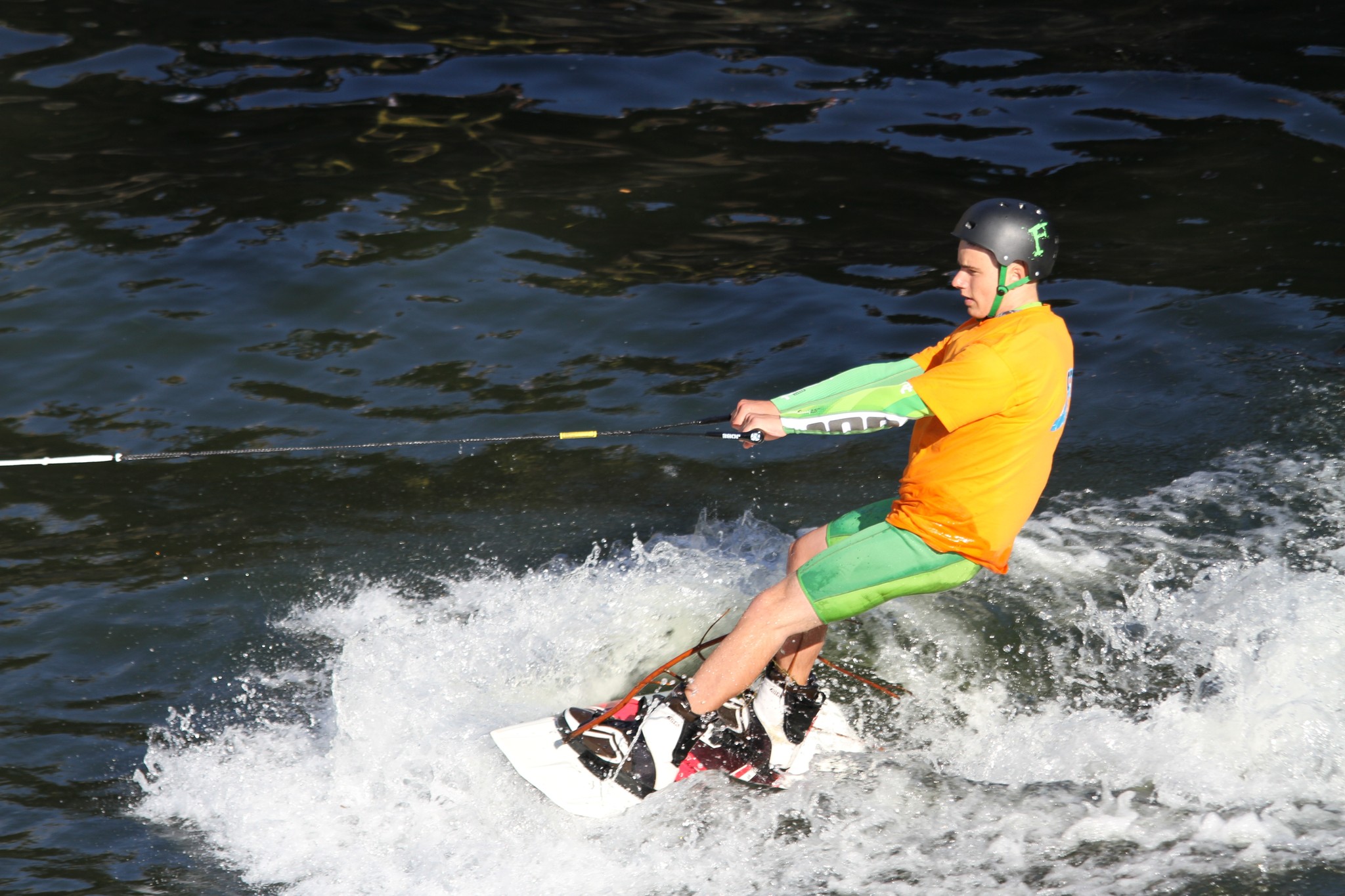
[490,691,869,821]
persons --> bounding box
[557,198,1073,791]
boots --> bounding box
[559,684,716,788]
[711,652,817,745]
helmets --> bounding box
[960,198,1058,281]
